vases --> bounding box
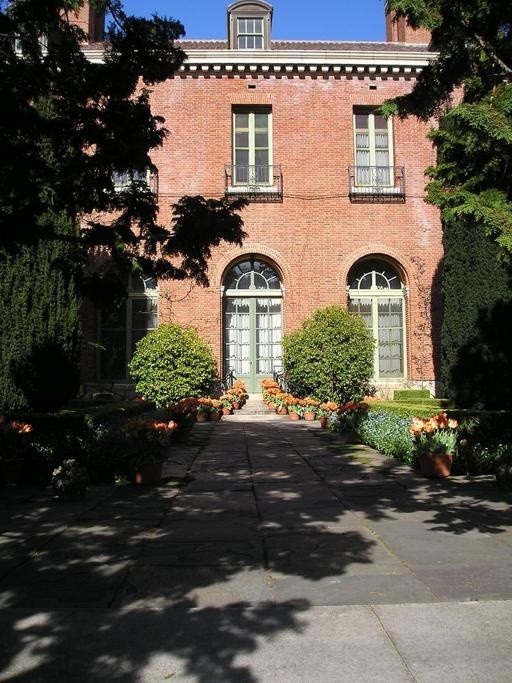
[419,451,453,477]
[345,428,360,443]
[126,454,162,484]
[196,408,230,421]
[289,411,328,428]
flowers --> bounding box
[121,419,179,454]
[51,459,76,490]
[196,378,247,412]
[336,400,369,430]
[260,377,336,418]
[411,412,468,454]
